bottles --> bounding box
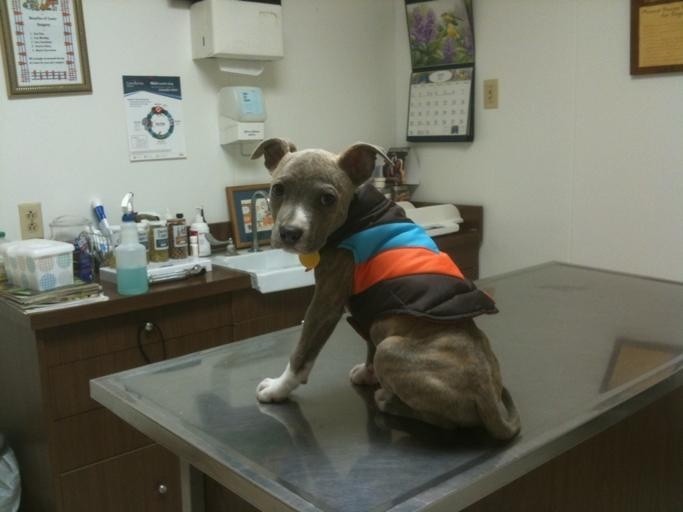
[190,207,212,260]
[148,218,198,263]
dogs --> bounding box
[249,136,523,448]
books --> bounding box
[0,274,107,315]
[369,174,466,238]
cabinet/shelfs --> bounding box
[1,229,481,511]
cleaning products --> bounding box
[116,192,148,296]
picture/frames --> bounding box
[630,2,682,75]
[1,1,92,100]
[224,183,274,249]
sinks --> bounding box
[212,247,318,295]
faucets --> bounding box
[248,188,271,252]
